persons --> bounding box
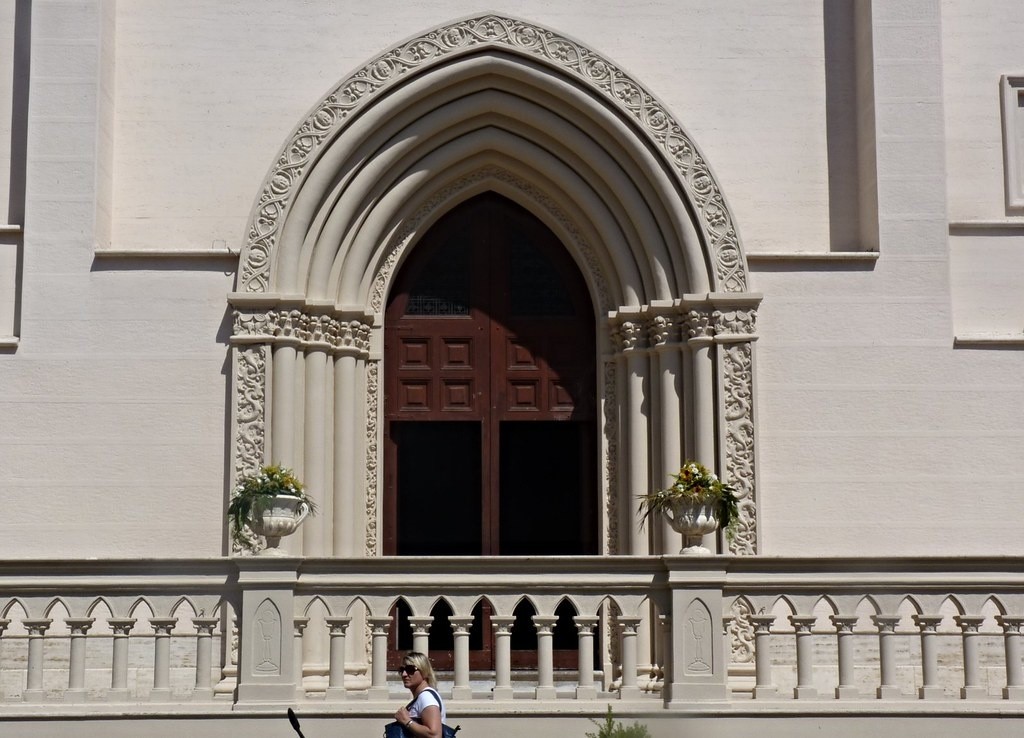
[383,652,461,738]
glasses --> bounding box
[398,665,419,675]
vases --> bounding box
[661,494,719,554]
[245,494,308,556]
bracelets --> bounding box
[405,720,413,729]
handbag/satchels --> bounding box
[384,690,461,738]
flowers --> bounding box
[633,460,739,539]
[226,463,318,547]
